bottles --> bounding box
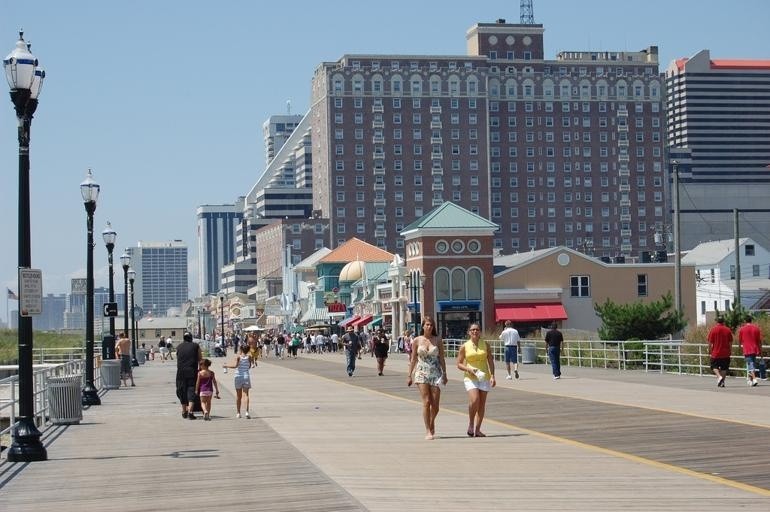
[223,361,229,373]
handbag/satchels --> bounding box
[758,354,766,379]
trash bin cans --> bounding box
[99,359,121,390]
[136,349,146,364]
[46,375,83,425]
[522,344,536,364]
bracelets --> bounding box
[408,375,411,377]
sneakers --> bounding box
[245,412,251,420]
[475,431,485,437]
[752,378,759,386]
[425,433,433,440]
[203,411,209,420]
[236,413,241,418]
[188,412,197,420]
[467,426,474,436]
[182,411,187,418]
[717,376,724,388]
[720,381,725,387]
[251,348,384,376]
[505,375,512,380]
[553,375,560,380]
[514,369,519,379]
[747,380,753,386]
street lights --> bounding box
[100,217,118,358]
[2,29,54,462]
[78,168,101,405]
[121,251,131,339]
[127,266,139,366]
[219,289,226,347]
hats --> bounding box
[346,326,355,332]
[160,336,165,338]
[715,315,726,323]
[167,335,173,338]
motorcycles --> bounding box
[218,346,228,357]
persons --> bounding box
[544,322,564,380]
[737,315,764,387]
[150,344,155,360]
[165,336,174,361]
[158,335,167,364]
[203,325,415,368]
[405,315,448,442]
[340,325,360,377]
[195,358,220,421]
[139,342,146,349]
[498,319,521,381]
[223,344,252,419]
[705,315,733,388]
[114,331,136,387]
[175,332,203,417]
[454,321,498,438]
[370,328,389,376]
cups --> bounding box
[381,338,384,343]
[349,340,352,346]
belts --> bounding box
[504,345,517,347]
